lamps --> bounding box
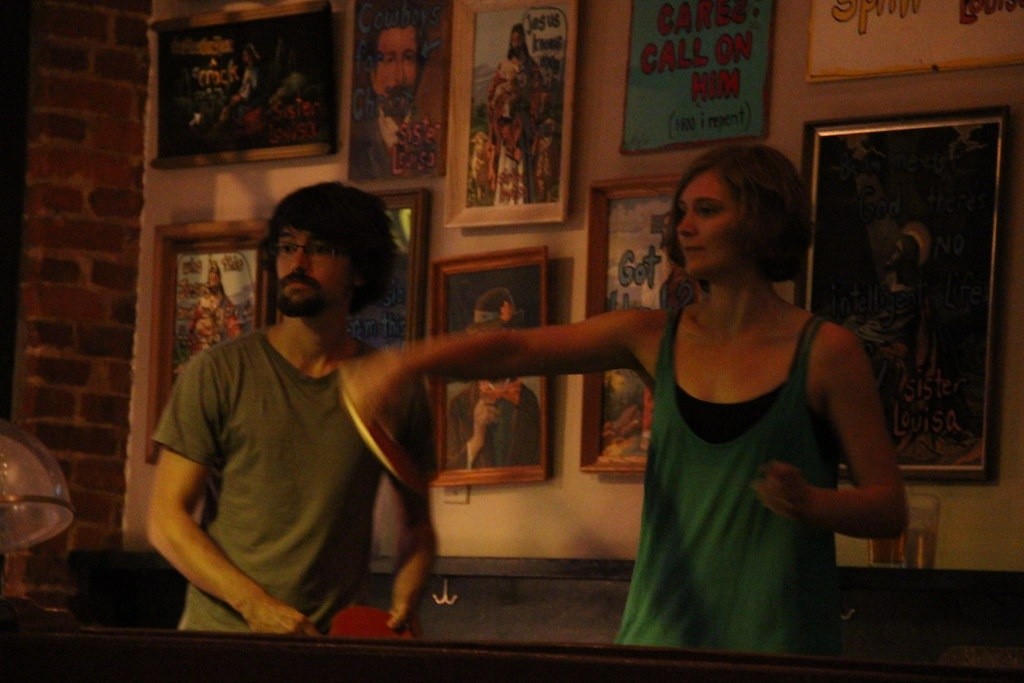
[0,419,78,554]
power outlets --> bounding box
[442,486,469,504]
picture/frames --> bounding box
[276,187,433,354]
[617,0,778,156]
[804,0,1024,83]
[795,104,1014,487]
[442,0,579,230]
[425,244,553,488]
[576,174,682,476]
[144,218,276,463]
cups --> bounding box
[903,492,941,568]
[868,538,904,569]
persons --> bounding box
[340,143,910,654]
[142,183,436,635]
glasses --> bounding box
[272,241,350,261]
